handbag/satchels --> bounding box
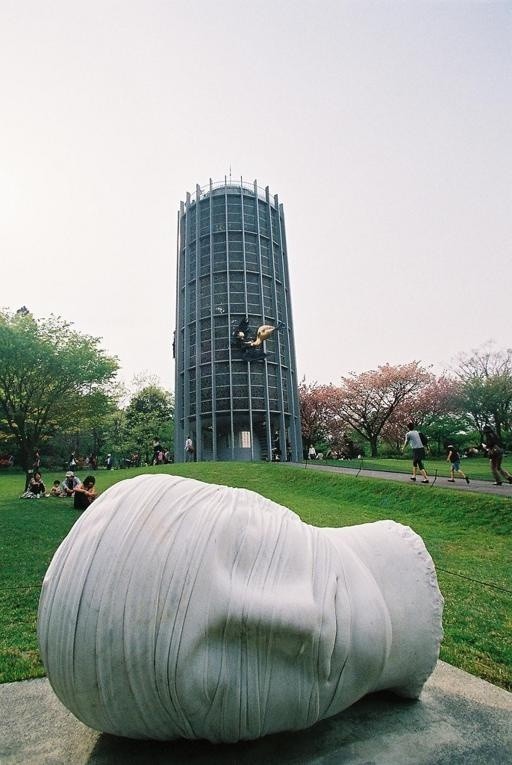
[488,444,503,456]
[418,433,429,445]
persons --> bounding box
[73,475,98,510]
[401,423,429,483]
[36,469,450,747]
[50,480,63,498]
[68,436,171,471]
[445,445,469,484]
[27,472,50,499]
[307,443,353,461]
[184,436,195,462]
[481,425,512,486]
[33,453,41,473]
[59,471,83,498]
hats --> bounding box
[65,471,74,477]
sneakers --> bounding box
[448,476,469,484]
[492,477,512,486]
[410,477,429,483]
